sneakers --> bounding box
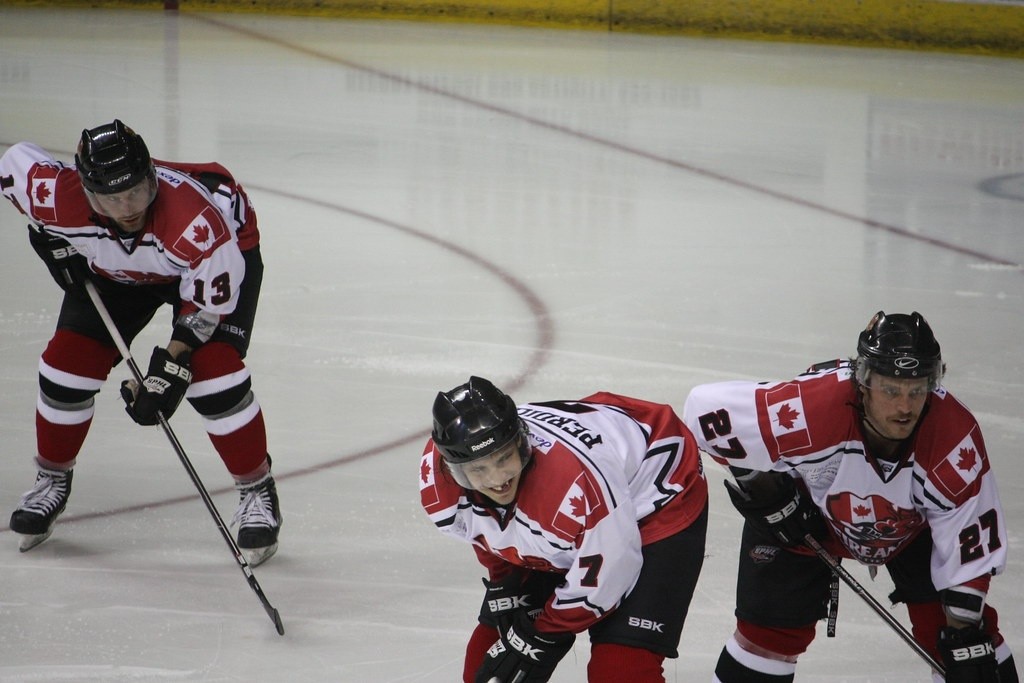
[227,452,283,568]
[9,456,74,553]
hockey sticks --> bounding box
[805,534,946,678]
[84,274,286,638]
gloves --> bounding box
[120,345,192,426]
[723,466,815,549]
[935,618,1000,683]
[472,576,576,683]
[27,224,102,294]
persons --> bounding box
[682,312,1018,682]
[0,119,280,568]
[419,375,708,683]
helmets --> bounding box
[430,374,531,465]
[856,309,942,380]
[74,118,152,196]
